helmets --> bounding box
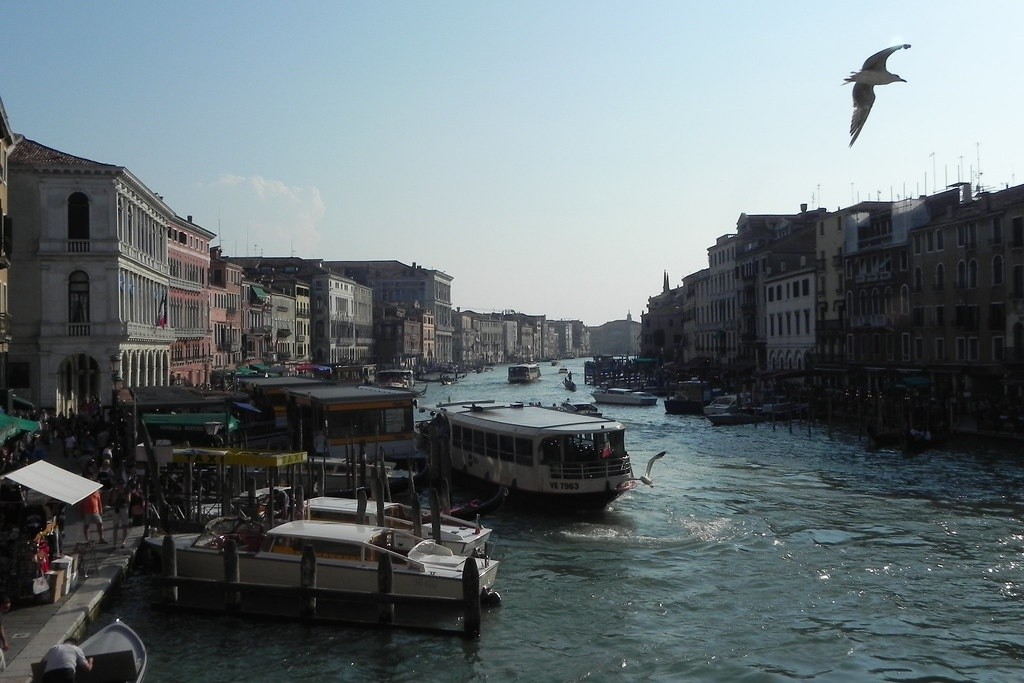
[103,459,110,465]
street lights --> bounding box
[108,353,120,511]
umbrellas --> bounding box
[297,361,315,373]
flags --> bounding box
[157,301,165,330]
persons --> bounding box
[0,596,10,672]
[130,470,186,520]
[108,480,132,550]
[40,637,93,683]
[273,489,289,519]
[78,490,109,543]
[0,407,116,506]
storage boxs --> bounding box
[41,553,81,603]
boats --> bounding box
[0,364,640,631]
[592,382,656,406]
[665,376,725,415]
[80,618,149,683]
[551,361,558,366]
[704,392,810,424]
[584,353,625,375]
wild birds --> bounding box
[615,450,667,489]
[840,43,911,151]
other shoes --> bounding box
[121,543,126,548]
[99,538,108,544]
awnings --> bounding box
[252,364,270,372]
[12,397,33,408]
[16,419,39,433]
[250,286,267,300]
